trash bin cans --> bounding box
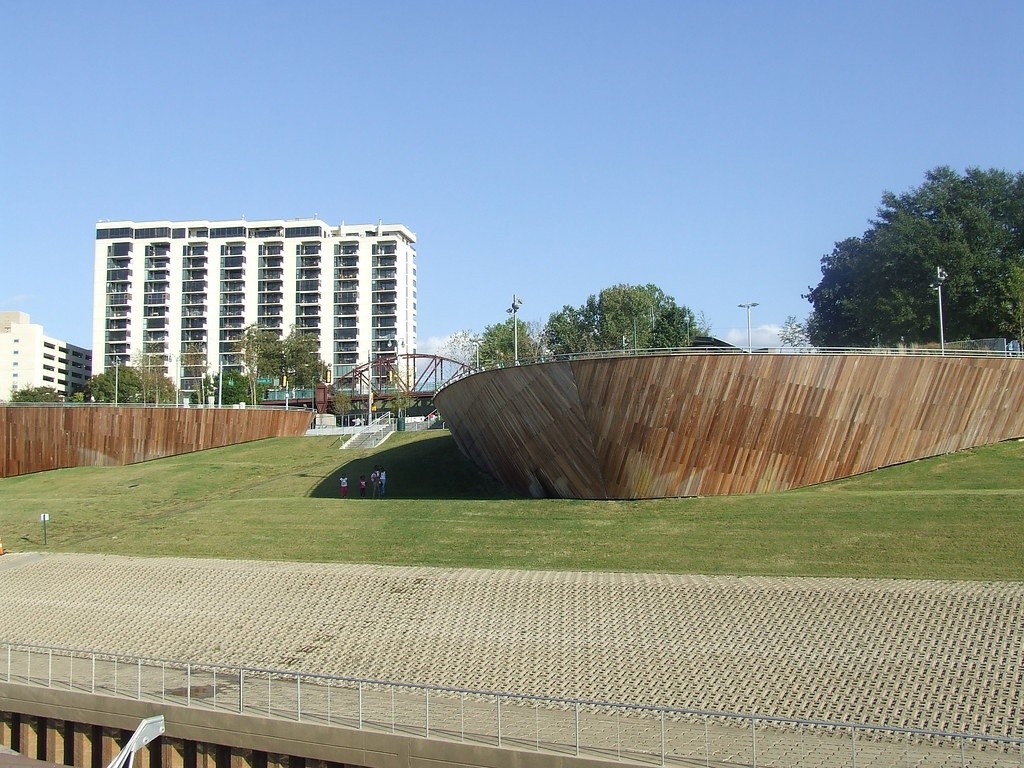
[398,417,405,431]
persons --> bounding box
[359,475,368,496]
[339,473,349,496]
[371,464,390,498]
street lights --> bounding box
[506,292,522,366]
[925,265,948,356]
[112,354,121,404]
[738,301,759,354]
